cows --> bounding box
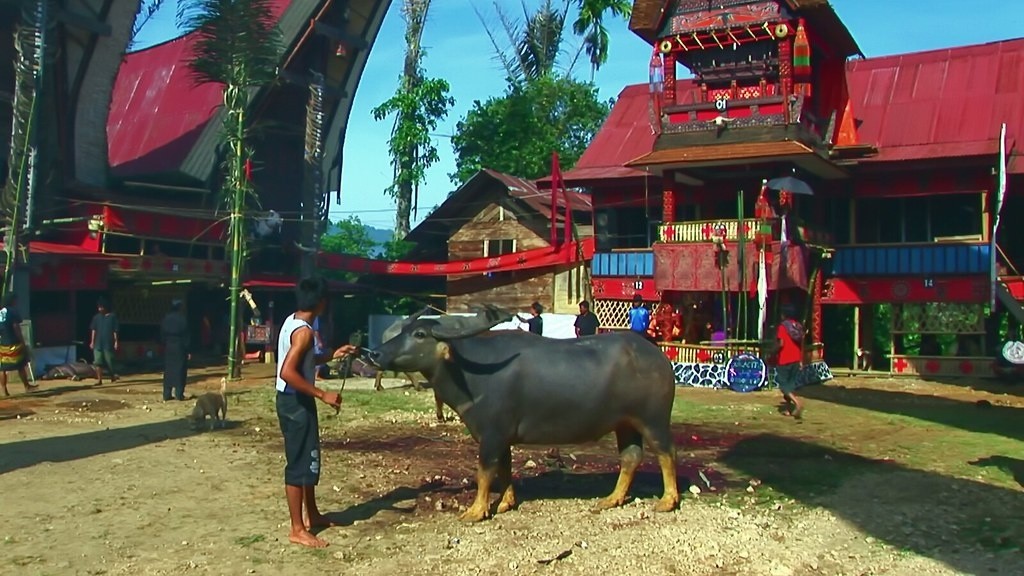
[367,302,681,523]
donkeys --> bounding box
[41,361,120,381]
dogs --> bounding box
[185,392,227,432]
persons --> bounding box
[573,301,600,338]
[628,294,650,335]
[1,291,44,397]
[772,303,805,418]
[516,300,544,335]
[162,297,193,401]
[275,272,363,545]
[90,297,118,385]
